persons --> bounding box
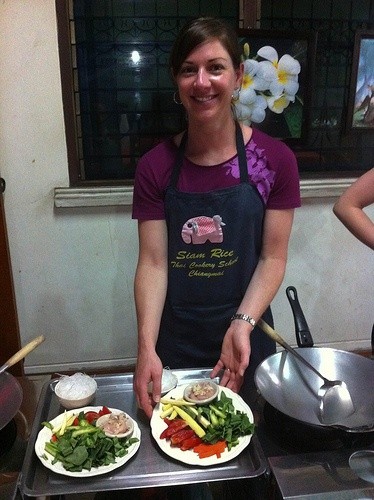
[132,15,302,418]
[333,167,374,356]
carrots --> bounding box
[193,439,227,458]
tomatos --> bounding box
[73,405,112,426]
[160,418,202,451]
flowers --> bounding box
[229,40,310,125]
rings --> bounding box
[225,368,229,371]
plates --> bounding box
[34,406,141,478]
[149,383,254,466]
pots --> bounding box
[0,370,23,431]
[255,286,374,434]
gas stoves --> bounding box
[0,373,53,500]
[238,367,374,500]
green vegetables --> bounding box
[41,411,140,472]
[171,390,257,451]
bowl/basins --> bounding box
[183,380,219,405]
[148,373,179,397]
[54,373,98,410]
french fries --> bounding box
[50,410,91,437]
[159,398,226,437]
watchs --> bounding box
[231,314,257,327]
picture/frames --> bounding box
[344,32,374,132]
[231,29,322,151]
